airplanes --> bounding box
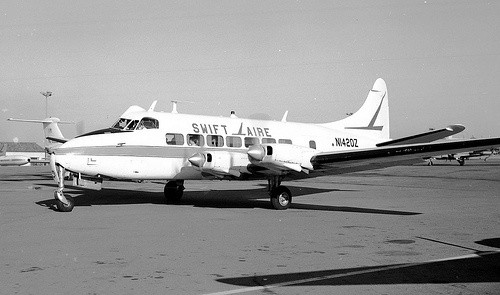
[421,149,500,166]
[50,78,500,212]
[0,117,76,181]
